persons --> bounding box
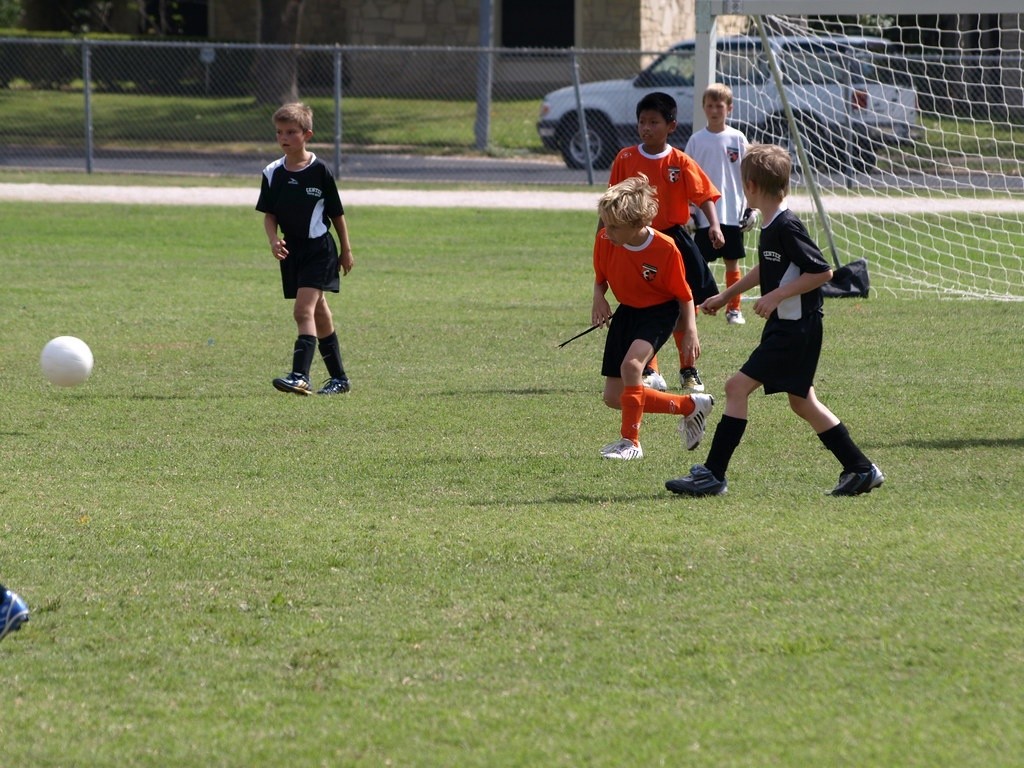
[255,104,355,395]
[0,584,29,644]
[665,145,885,496]
[592,177,714,460]
[683,83,760,324]
[596,92,725,393]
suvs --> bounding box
[534,34,926,175]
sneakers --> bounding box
[600,438,643,461]
[665,464,727,497]
[642,367,667,393]
[726,309,745,325]
[825,463,884,498]
[677,393,714,451]
[316,377,351,396]
[679,366,704,393]
[273,370,312,395]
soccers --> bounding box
[39,336,94,387]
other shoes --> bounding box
[0,590,28,641]
